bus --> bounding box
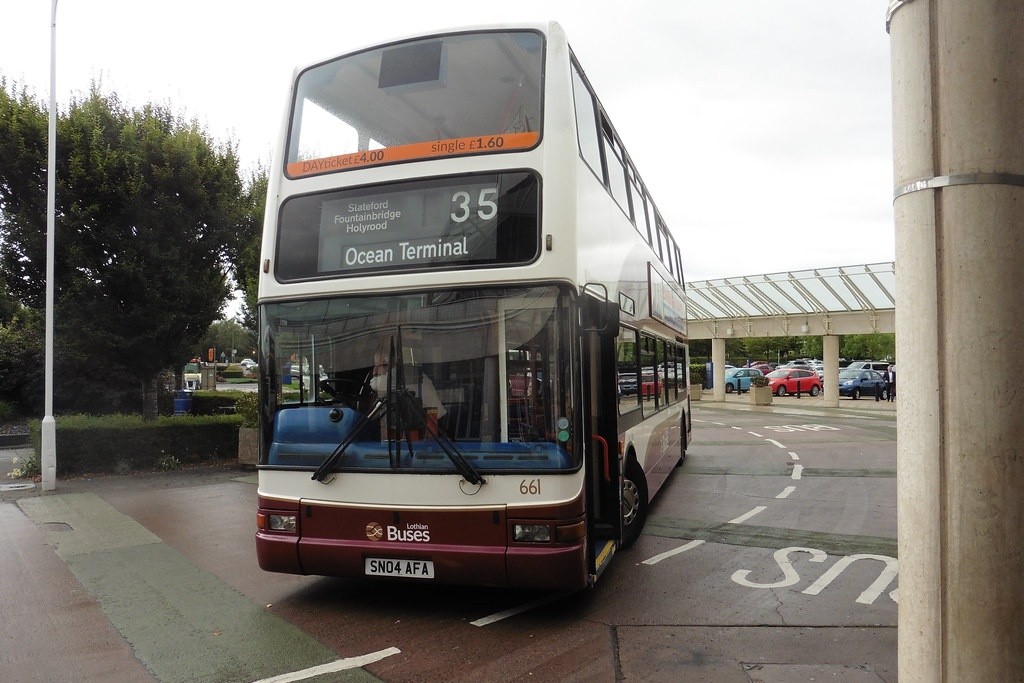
[174,359,203,389]
[248,18,690,606]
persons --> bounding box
[370,348,447,427]
[883,366,896,402]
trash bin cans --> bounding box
[173,392,192,417]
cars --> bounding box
[617,362,688,400]
[724,357,896,401]
[241,358,257,369]
[290,362,326,380]
